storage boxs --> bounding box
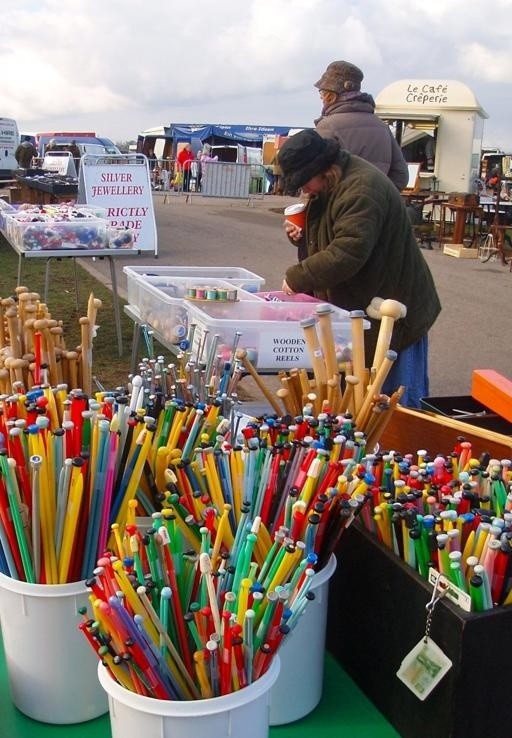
[123,265,371,372]
[1,197,136,250]
[481,159,489,178]
[255,393,512,737]
[448,192,480,206]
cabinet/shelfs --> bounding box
[400,189,446,246]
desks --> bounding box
[14,173,78,204]
[438,201,483,248]
[0,228,141,358]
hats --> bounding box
[313,61,363,94]
[278,127,340,190]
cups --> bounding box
[284,204,306,238]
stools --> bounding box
[481,224,512,272]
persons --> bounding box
[424,128,439,173]
[277,127,442,410]
[311,60,410,193]
[14,136,222,192]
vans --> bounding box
[40,136,123,157]
[0,116,19,187]
